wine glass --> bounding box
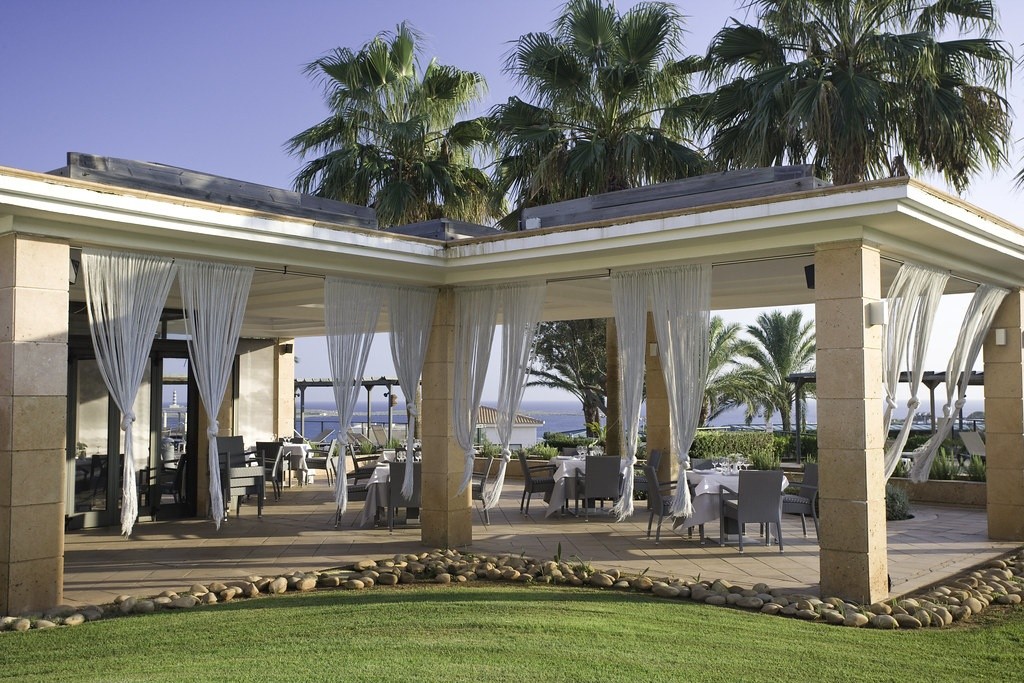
[710,454,751,471]
[397,451,422,463]
[271,433,292,443]
[576,445,604,461]
[400,439,422,450]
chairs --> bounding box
[74,435,818,554]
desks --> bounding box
[544,457,612,520]
[283,442,310,488]
[685,468,787,545]
